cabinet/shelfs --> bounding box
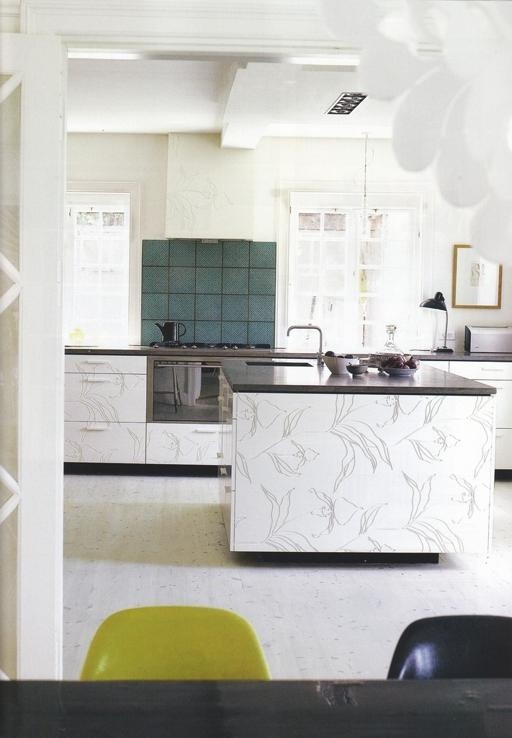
[146,423,220,478]
[448,359,512,481]
[63,354,147,476]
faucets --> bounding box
[286,325,324,364]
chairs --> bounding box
[387,615,511,680]
[80,604,269,684]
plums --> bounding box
[382,356,417,369]
[325,351,354,358]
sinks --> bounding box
[245,360,313,367]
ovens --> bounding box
[146,358,232,423]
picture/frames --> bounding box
[452,245,502,310]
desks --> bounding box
[0,678,512,737]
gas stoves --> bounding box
[150,340,272,349]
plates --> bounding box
[378,367,419,378]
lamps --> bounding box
[419,290,454,353]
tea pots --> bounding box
[154,320,186,345]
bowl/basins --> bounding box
[320,353,360,375]
[347,365,368,377]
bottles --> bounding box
[377,325,406,375]
